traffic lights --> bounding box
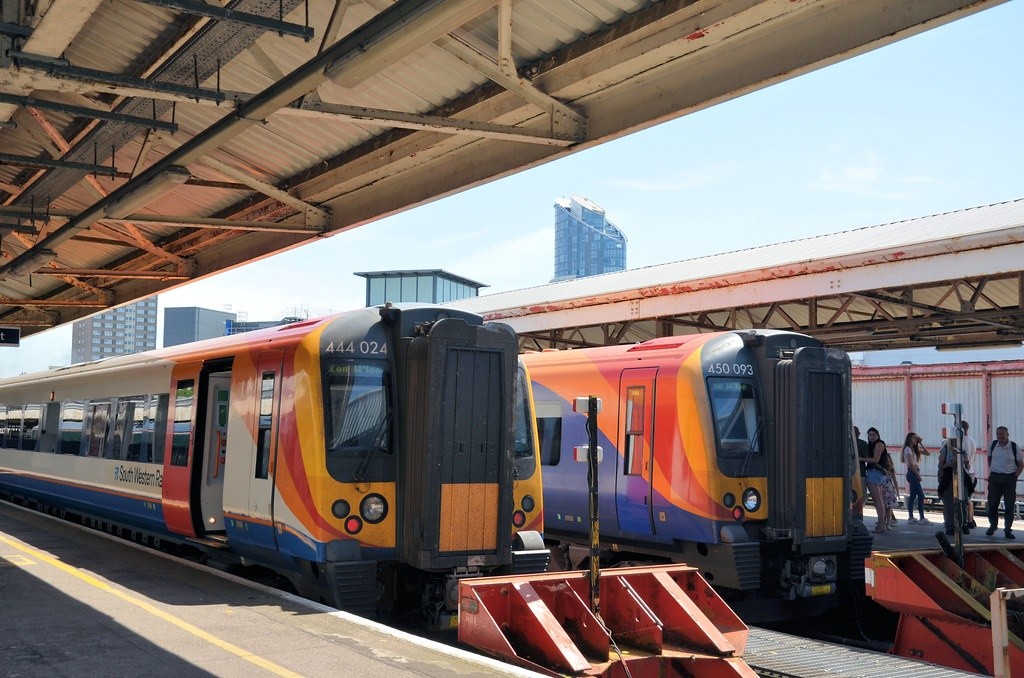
[941,403,956,440]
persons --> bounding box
[986,426,1024,539]
[901,432,930,524]
[938,421,978,534]
[854,426,899,532]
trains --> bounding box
[0,302,551,642]
[517,330,875,607]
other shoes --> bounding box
[908,517,916,524]
[986,524,998,535]
[919,520,930,525]
[946,529,954,535]
[964,527,970,535]
[967,522,974,529]
[872,518,897,533]
[1005,531,1015,539]
